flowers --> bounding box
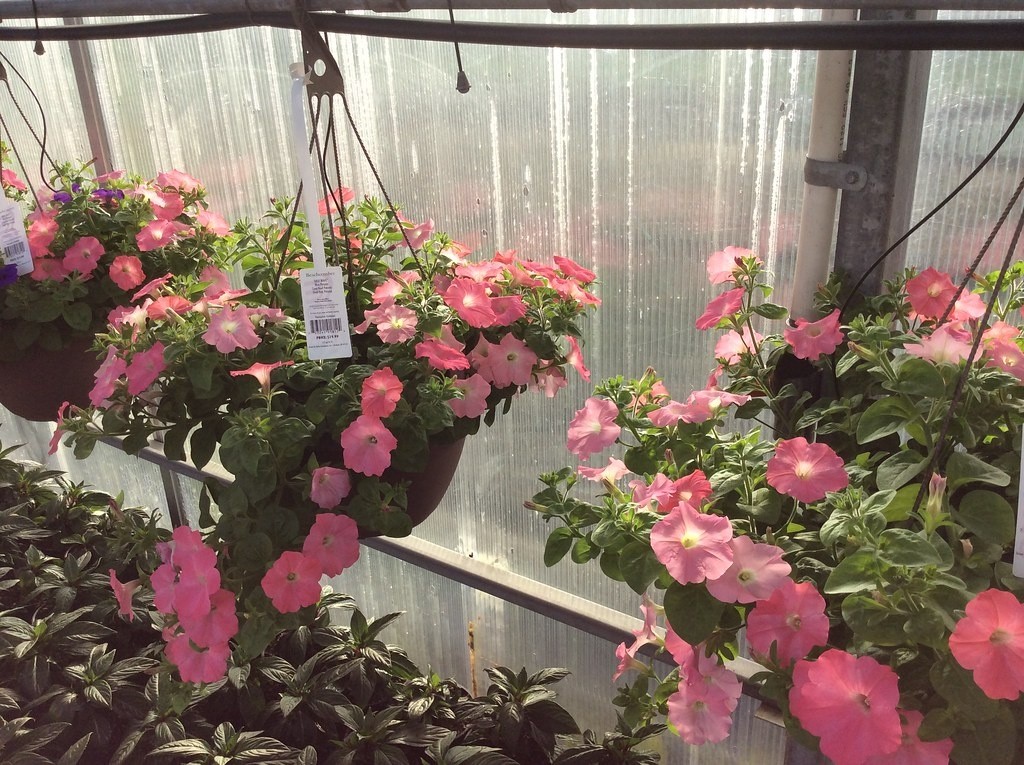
[0,140,1024,765]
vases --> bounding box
[287,403,465,539]
[1,321,126,421]
